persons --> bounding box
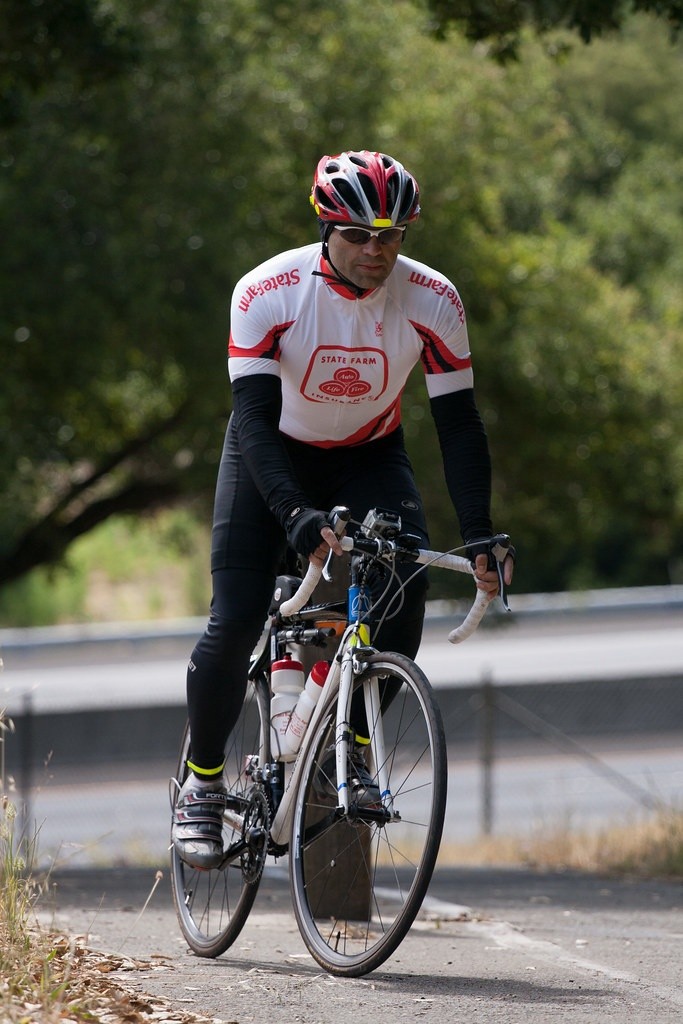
[173,150,515,869]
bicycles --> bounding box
[169,507,511,977]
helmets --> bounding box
[309,149,421,229]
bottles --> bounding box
[270,652,305,762]
[284,660,330,752]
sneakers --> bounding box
[171,772,227,869]
[313,746,380,804]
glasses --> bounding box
[334,225,406,245]
